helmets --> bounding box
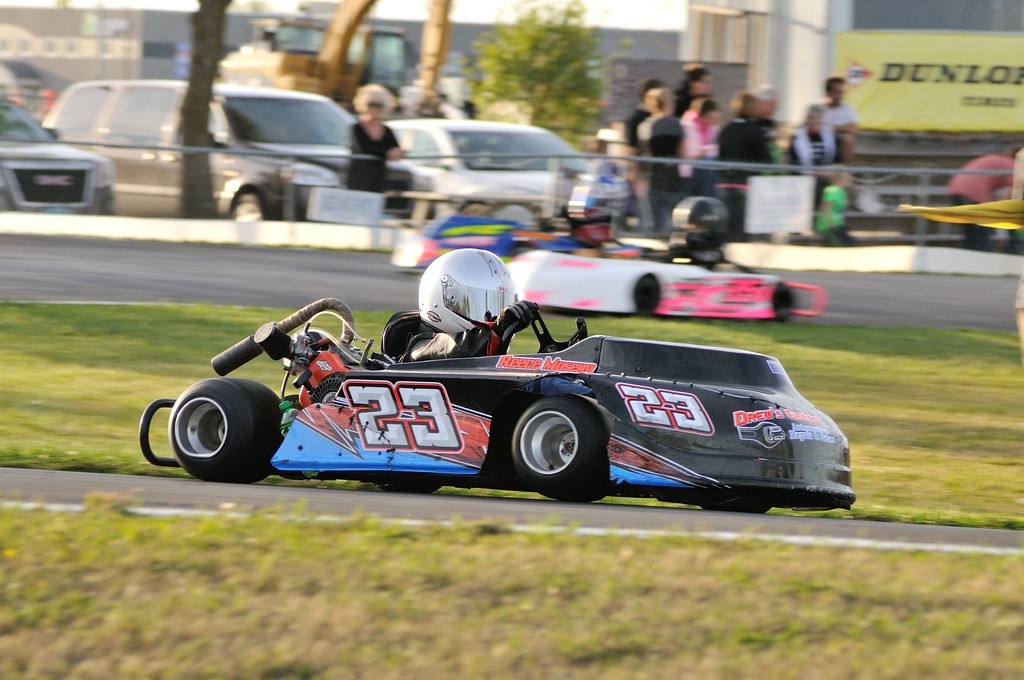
[416,249,518,354]
[671,194,732,244]
[558,171,631,229]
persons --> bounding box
[949,147,1020,254]
[347,84,411,191]
[566,173,631,245]
[670,194,729,266]
[623,62,861,242]
[404,247,539,360]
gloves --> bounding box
[490,298,537,341]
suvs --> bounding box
[0,89,115,220]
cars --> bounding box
[379,117,629,243]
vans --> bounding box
[44,79,364,213]
